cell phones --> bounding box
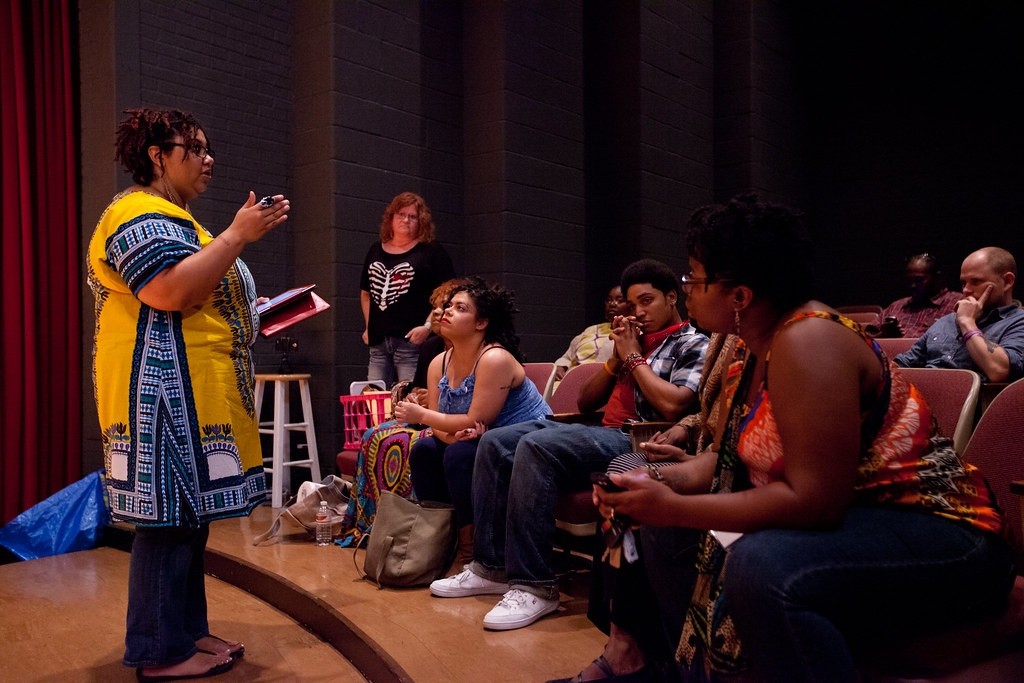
[885,316,899,325]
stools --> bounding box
[252,374,322,507]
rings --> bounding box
[260,196,274,208]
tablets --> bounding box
[256,283,316,315]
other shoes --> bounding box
[548,655,651,683]
[136,659,235,683]
[196,634,244,658]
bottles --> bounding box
[316,501,332,546]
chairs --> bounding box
[337,305,1024,683]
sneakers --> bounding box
[431,565,510,597]
[483,589,559,630]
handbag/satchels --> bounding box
[353,490,454,589]
[253,475,352,546]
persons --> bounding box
[395,277,554,568]
[555,285,632,382]
[430,260,710,634]
[894,247,1024,425]
[557,195,1019,683]
[879,247,963,338]
[361,192,455,391]
[86,109,290,683]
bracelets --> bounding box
[645,463,665,484]
[624,352,647,373]
[605,362,615,375]
[963,328,982,343]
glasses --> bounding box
[394,211,419,222]
[682,274,725,284]
[170,143,216,158]
